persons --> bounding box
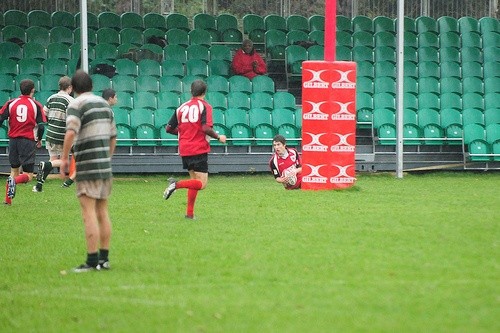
[0,79,48,206]
[269,135,303,190]
[60,88,117,188]
[31,76,75,193]
[60,70,118,272]
[233,39,268,80]
[163,80,227,218]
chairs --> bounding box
[0,10,500,168]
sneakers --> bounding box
[32,185,42,192]
[61,183,69,187]
[7,176,16,199]
[36,162,53,183]
[163,177,177,200]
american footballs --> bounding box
[282,169,297,186]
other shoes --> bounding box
[98,259,109,269]
[73,264,100,272]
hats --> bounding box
[242,40,254,48]
